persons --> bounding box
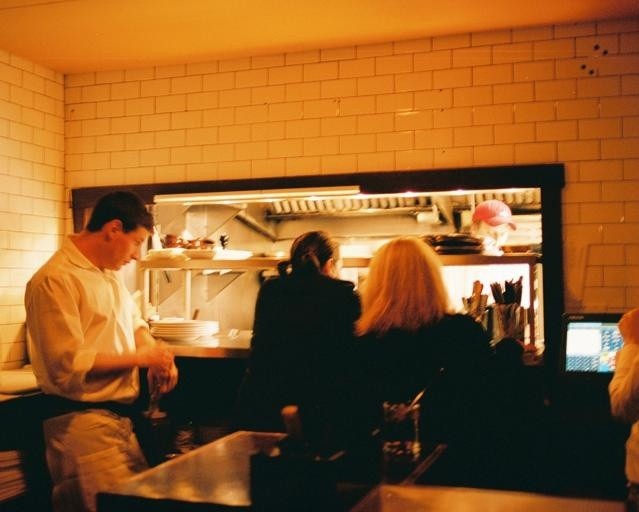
[336,236,526,491]
[608,308,639,504]
[249,230,363,430]
[24,190,179,512]
[470,200,516,251]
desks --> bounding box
[96,428,447,512]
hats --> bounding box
[469,199,521,232]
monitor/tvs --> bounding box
[560,311,623,381]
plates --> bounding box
[149,321,219,341]
[148,248,185,258]
[183,250,253,259]
